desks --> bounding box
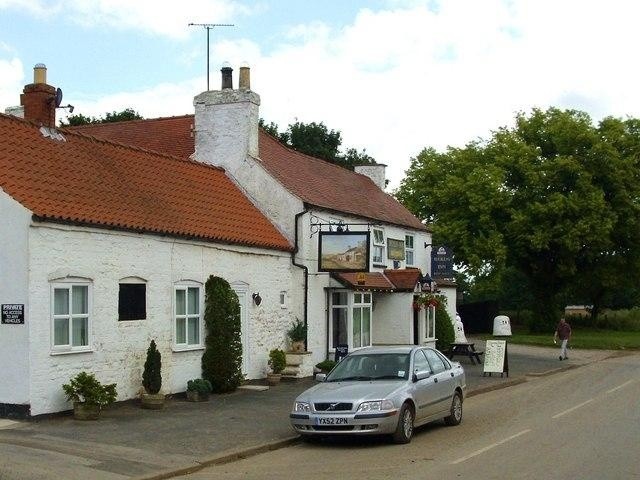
[449,343,482,365]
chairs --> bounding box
[351,358,376,377]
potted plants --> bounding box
[266,348,286,386]
[62,372,118,420]
[186,379,213,402]
[315,358,339,375]
[286,316,309,353]
[140,339,165,410]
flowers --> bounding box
[413,294,448,312]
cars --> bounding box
[290,345,467,445]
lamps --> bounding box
[252,292,262,305]
[424,242,437,248]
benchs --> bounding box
[442,348,450,359]
[472,350,485,357]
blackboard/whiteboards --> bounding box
[483,339,508,373]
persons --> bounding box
[554,314,571,360]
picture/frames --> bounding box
[318,231,370,273]
[387,237,405,260]
